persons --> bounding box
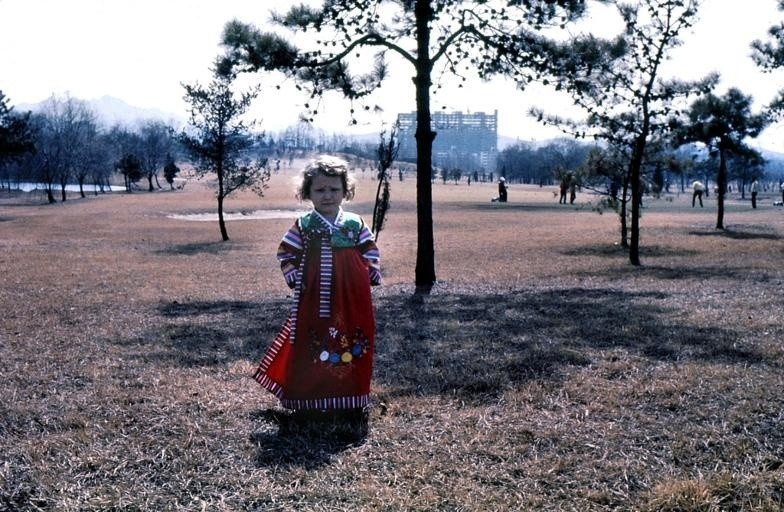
[250,152,385,421]
[492,174,509,203]
[691,179,705,209]
[569,176,578,204]
[559,179,570,204]
[750,175,759,210]
